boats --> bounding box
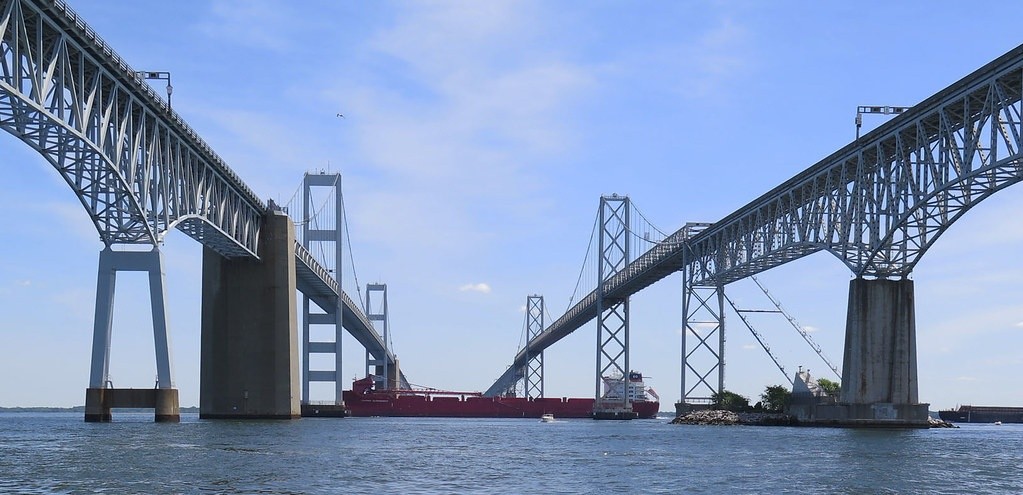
[343,372,659,422]
[542,413,554,423]
[994,422,1001,425]
[938,406,1023,423]
